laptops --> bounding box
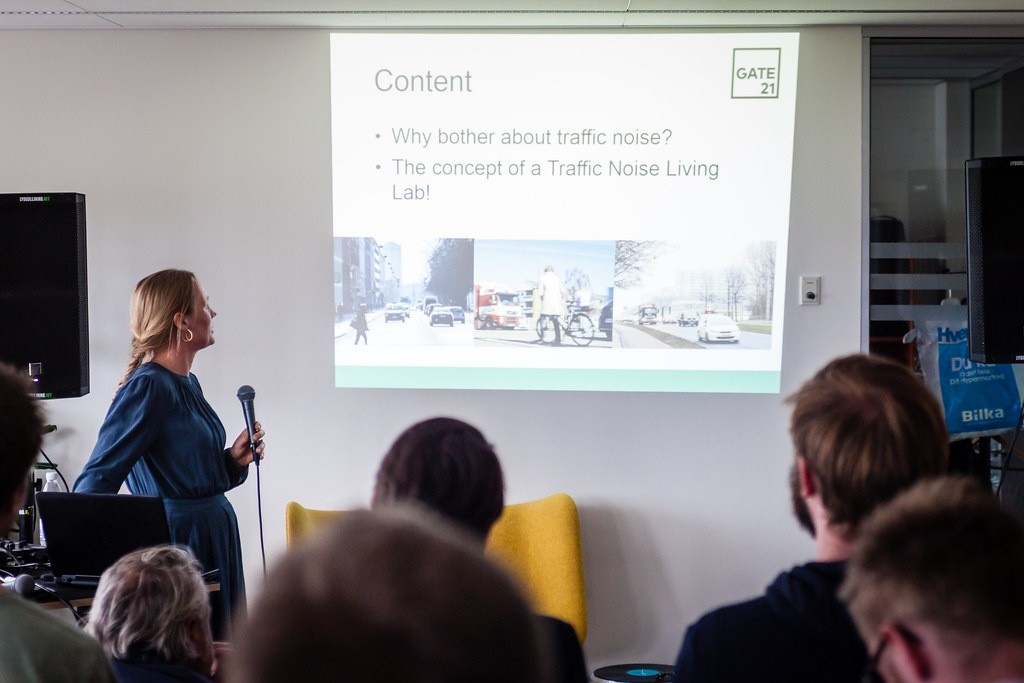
[34,491,220,586]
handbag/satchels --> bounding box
[350,317,358,330]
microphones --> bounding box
[0,574,35,595]
[236,385,260,467]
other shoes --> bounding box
[550,339,560,346]
[535,340,544,345]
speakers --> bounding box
[0,192,91,400]
[965,155,1024,364]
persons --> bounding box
[372,412,596,683]
[531,265,568,344]
[350,303,369,345]
[70,269,266,683]
[84,544,215,683]
[839,478,1024,683]
[233,512,550,683]
[0,361,114,683]
[675,353,987,683]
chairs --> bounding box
[283,492,590,646]
